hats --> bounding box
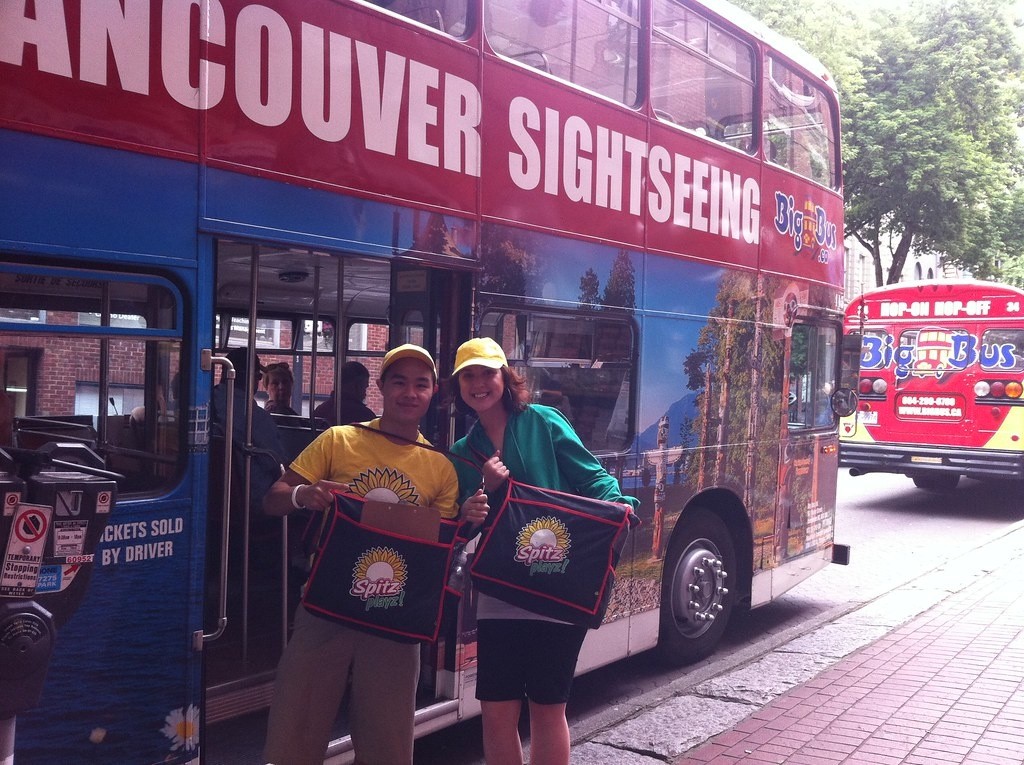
[452,336,508,376]
[382,344,435,374]
[223,347,267,374]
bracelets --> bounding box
[291,483,307,510]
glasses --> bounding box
[266,362,292,381]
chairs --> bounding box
[0,408,335,526]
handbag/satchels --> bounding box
[468,483,638,630]
[300,493,463,646]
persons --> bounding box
[214,347,289,463]
[167,372,181,411]
[260,344,461,765]
[262,362,303,427]
[313,360,377,428]
[443,337,642,765]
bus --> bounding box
[840,279,1024,490]
[0,0,852,765]
